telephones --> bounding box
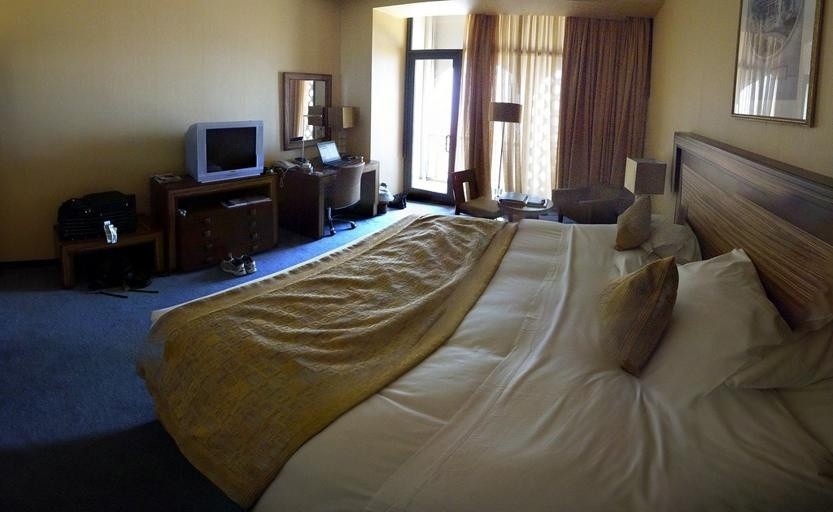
[272,159,297,170]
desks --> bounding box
[149,173,278,275]
[278,162,379,239]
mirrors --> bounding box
[284,71,332,150]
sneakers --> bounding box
[220,257,247,276]
[232,254,257,274]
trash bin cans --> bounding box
[377,188,390,214]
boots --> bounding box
[388,193,406,209]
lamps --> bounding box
[488,102,522,200]
[327,106,360,130]
[624,157,667,195]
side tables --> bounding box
[59,217,164,286]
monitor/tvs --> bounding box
[183,120,264,184]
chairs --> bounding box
[449,168,502,220]
[552,185,635,224]
[326,162,365,235]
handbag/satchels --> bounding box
[53,191,137,242]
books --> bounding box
[499,192,548,208]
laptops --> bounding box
[316,140,357,168]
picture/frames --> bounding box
[731,0,822,127]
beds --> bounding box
[136,132,833,512]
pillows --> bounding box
[599,197,790,394]
